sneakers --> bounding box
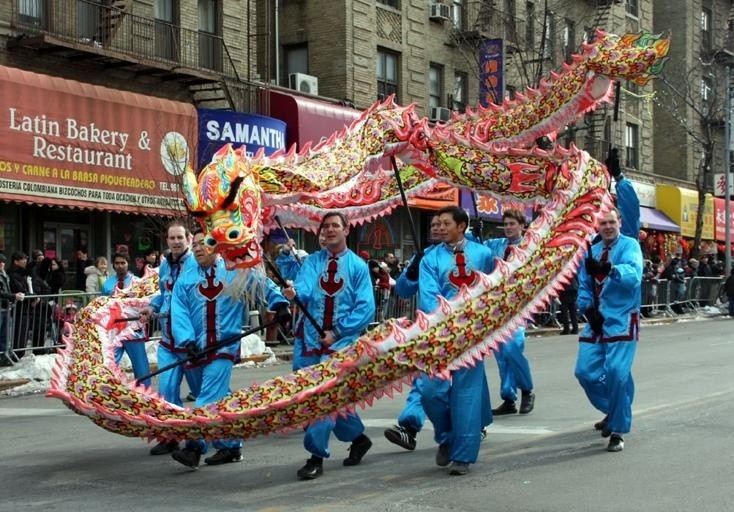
[448,461,466,475]
[595,416,624,451]
[204,447,242,464]
[491,401,517,414]
[172,448,200,470]
[151,440,178,455]
[343,436,373,466]
[520,391,535,413]
[436,444,451,466]
[384,428,416,450]
[297,459,322,479]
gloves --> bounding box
[605,148,620,176]
[187,344,204,363]
[407,253,423,279]
[585,258,610,274]
[276,304,291,323]
[586,308,603,333]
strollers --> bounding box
[47,286,87,353]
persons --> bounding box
[102,252,153,400]
[575,209,644,451]
[393,210,494,453]
[1,246,171,361]
[530,274,579,335]
[477,209,535,413]
[281,213,376,480]
[171,227,290,470]
[361,251,416,322]
[250,267,299,345]
[592,147,641,439]
[274,218,327,432]
[138,222,210,457]
[419,206,495,478]
[643,254,733,317]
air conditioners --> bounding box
[288,72,318,95]
[431,107,450,121]
[431,4,450,19]
